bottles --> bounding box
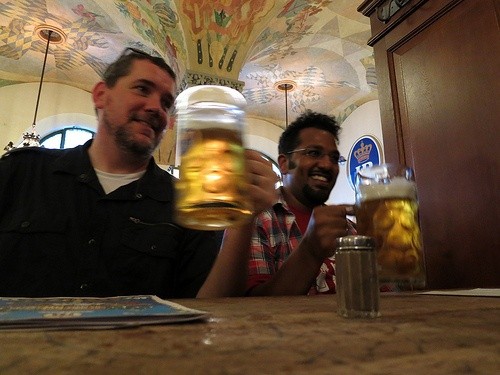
[333,234,381,318]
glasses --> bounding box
[285,147,347,164]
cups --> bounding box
[358,182,427,293]
[170,83,255,232]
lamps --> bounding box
[4,30,54,152]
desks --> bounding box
[0,288,500,375]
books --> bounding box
[0,295,212,331]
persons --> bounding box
[0,48,278,299]
[221,112,396,296]
